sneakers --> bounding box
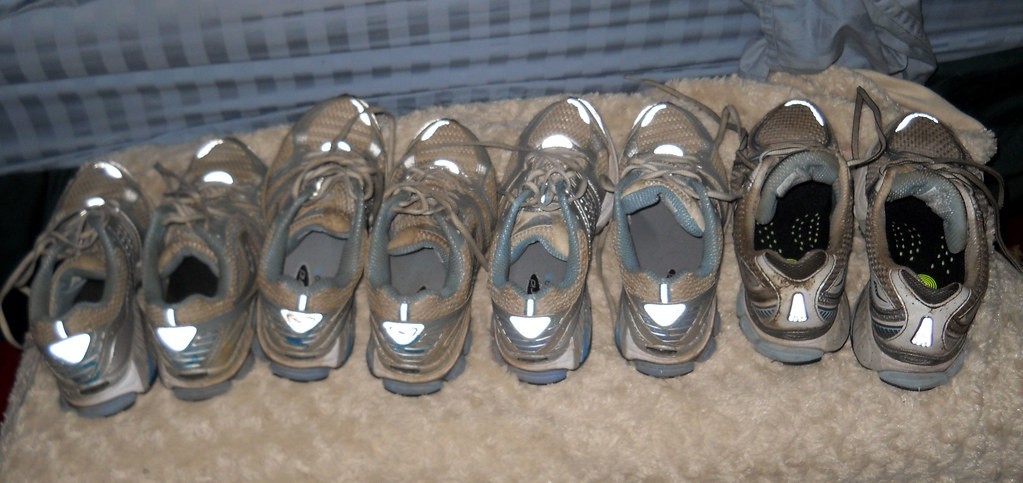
[0,154,158,418]
[251,93,395,382]
[486,96,619,385]
[599,101,733,379]
[363,117,498,398]
[642,77,854,365]
[137,136,270,402]
[851,86,1022,392]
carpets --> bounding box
[0,62,1023,483]
